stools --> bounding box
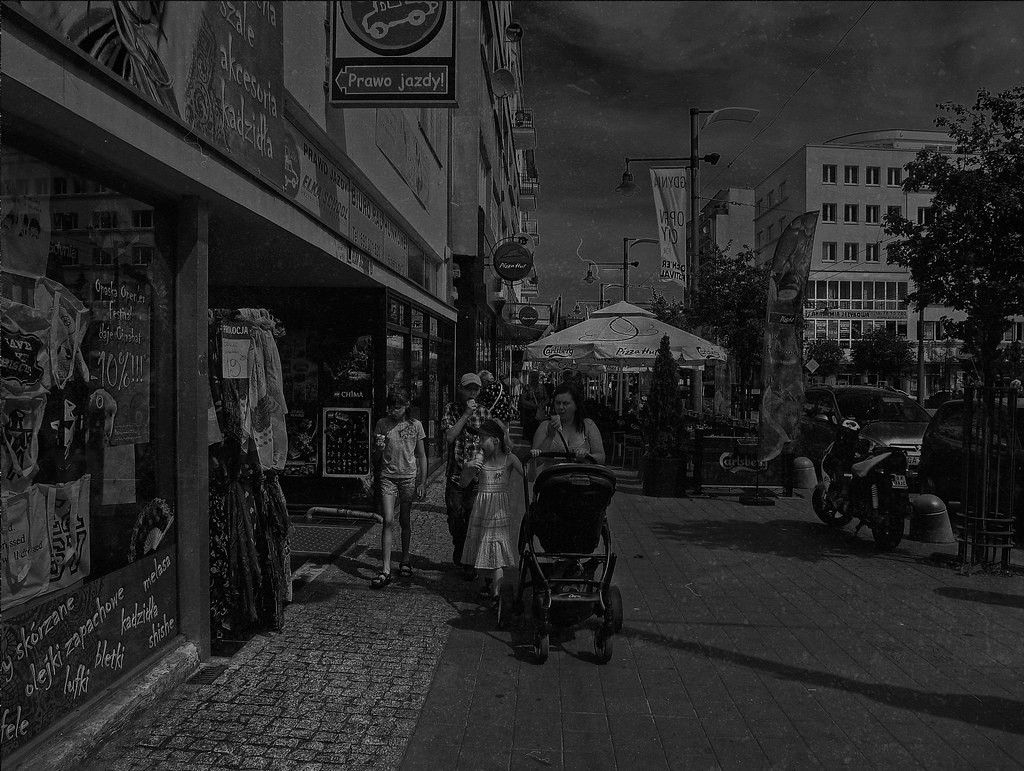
[611,431,625,471]
[622,436,641,470]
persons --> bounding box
[441,373,492,566]
[371,388,427,586]
[531,381,605,488]
[1010,379,1024,399]
[459,418,542,602]
[478,369,515,440]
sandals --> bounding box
[372,571,390,589]
[399,561,412,580]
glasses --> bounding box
[385,405,404,412]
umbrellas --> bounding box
[523,302,728,418]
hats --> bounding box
[466,419,504,443]
[460,373,482,388]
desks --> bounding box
[689,435,791,496]
[693,428,786,497]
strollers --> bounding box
[498,449,625,663]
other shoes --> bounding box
[479,585,489,598]
[465,565,478,581]
[491,595,499,609]
[453,545,462,569]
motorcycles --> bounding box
[811,413,916,550]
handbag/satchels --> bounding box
[0,278,92,611]
[534,407,546,422]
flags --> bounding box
[649,167,688,288]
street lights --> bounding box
[617,108,761,493]
[571,283,632,314]
[585,237,660,304]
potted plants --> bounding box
[637,331,690,499]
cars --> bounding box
[923,390,965,417]
[896,390,917,400]
[921,398,1023,543]
[683,382,716,415]
[802,386,934,479]
